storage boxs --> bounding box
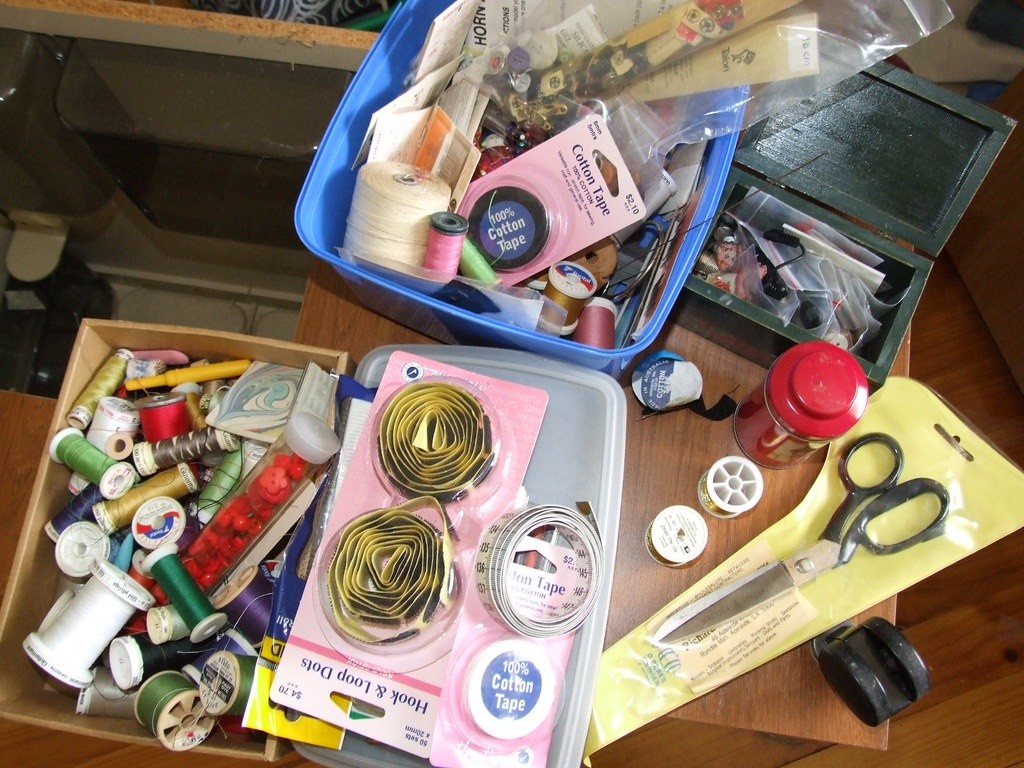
[1,315,354,762]
[294,0,751,382]
[54,37,358,250]
[290,344,628,768]
[666,42,1019,396]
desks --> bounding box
[294,251,911,767]
[0,387,328,767]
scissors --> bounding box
[650,432,951,649]
